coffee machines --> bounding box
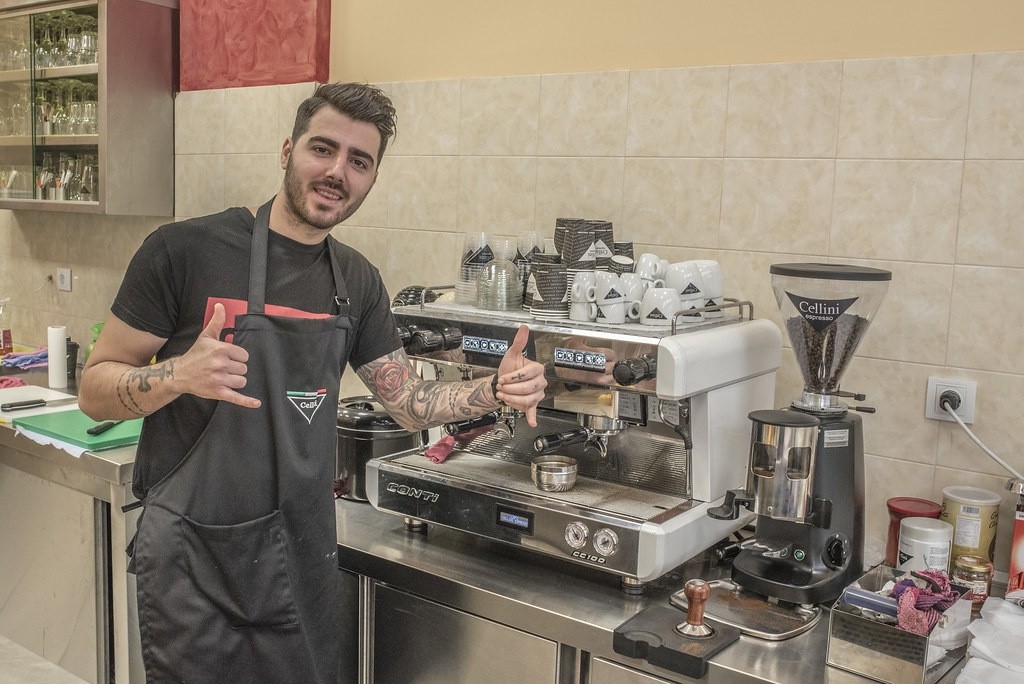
[708,262,893,604]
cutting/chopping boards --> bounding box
[12,408,145,452]
[0,384,80,423]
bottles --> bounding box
[35,151,99,202]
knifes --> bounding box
[88,420,124,435]
[1,396,79,411]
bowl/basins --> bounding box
[530,455,578,492]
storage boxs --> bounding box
[825,565,973,684]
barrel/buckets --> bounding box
[334,394,421,503]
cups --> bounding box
[454,217,725,326]
[37,185,66,201]
[67,342,81,381]
[65,31,95,65]
[42,122,52,135]
[66,99,97,133]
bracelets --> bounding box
[491,374,509,406]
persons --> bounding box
[460,323,616,384]
[78,84,547,684]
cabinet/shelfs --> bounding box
[336,496,779,684]
[0,363,147,684]
[1,0,175,218]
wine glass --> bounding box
[14,79,99,136]
[4,12,98,73]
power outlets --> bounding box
[925,377,977,424]
[57,268,72,291]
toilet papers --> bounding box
[46,326,68,388]
